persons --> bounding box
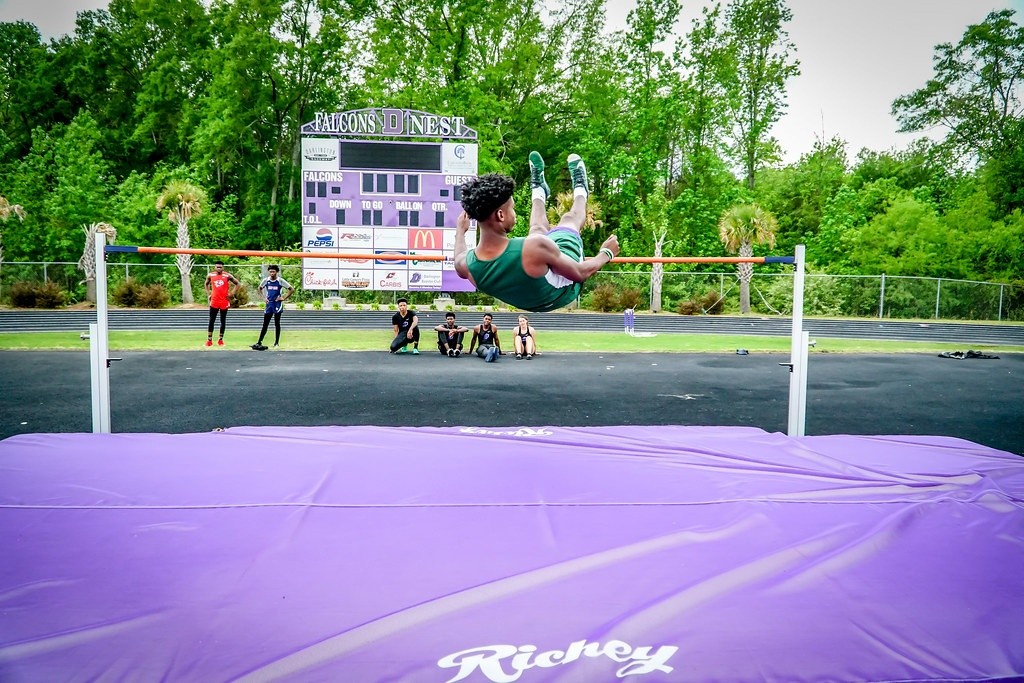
[203,261,241,346]
[434,313,469,357]
[389,298,420,354]
[248,264,295,346]
[508,314,542,360]
[453,150,620,313]
[464,313,506,362]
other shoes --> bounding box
[218,340,223,345]
[526,353,532,360]
[495,346,499,360]
[256,343,260,348]
[454,349,461,357]
[447,348,454,357]
[207,341,212,346]
[401,345,408,352]
[413,348,419,354]
[274,344,278,347]
[516,353,523,359]
[485,347,495,362]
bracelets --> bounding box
[600,248,614,264]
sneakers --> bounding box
[529,151,551,199]
[567,153,588,198]
[938,351,964,359]
